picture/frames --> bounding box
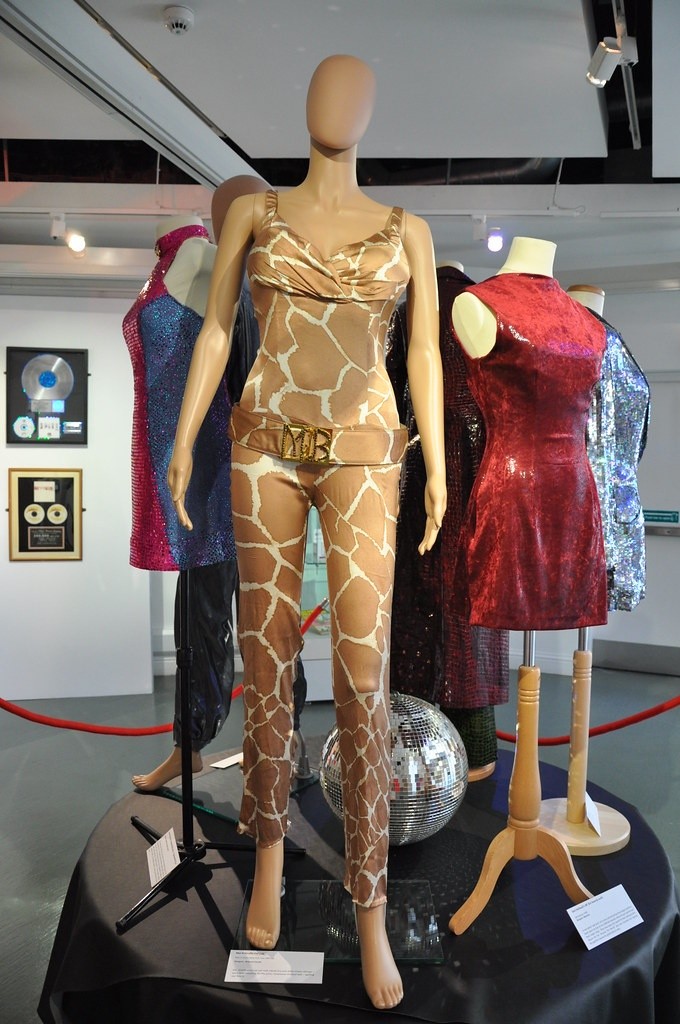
[8,467,83,561]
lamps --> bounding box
[585,37,626,89]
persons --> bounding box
[567,284,652,611]
[165,54,449,1009]
[387,260,512,777]
[449,236,610,630]
[121,175,310,792]
